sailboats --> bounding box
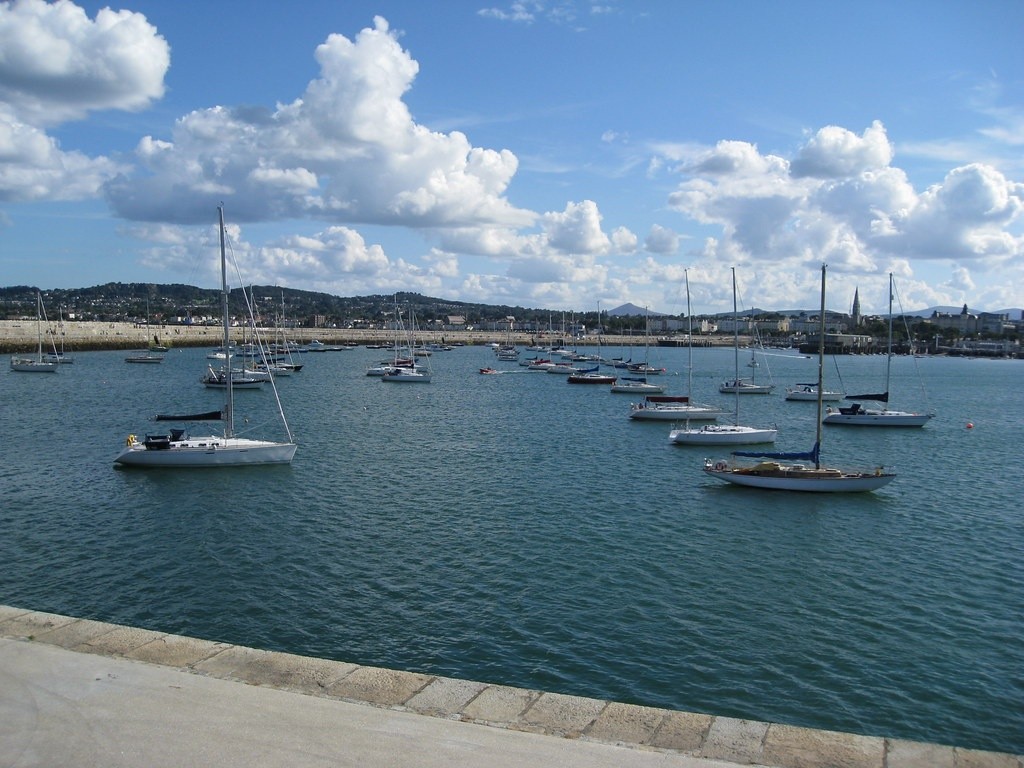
[703,263,897,493]
[10,291,73,372]
[113,199,938,466]
